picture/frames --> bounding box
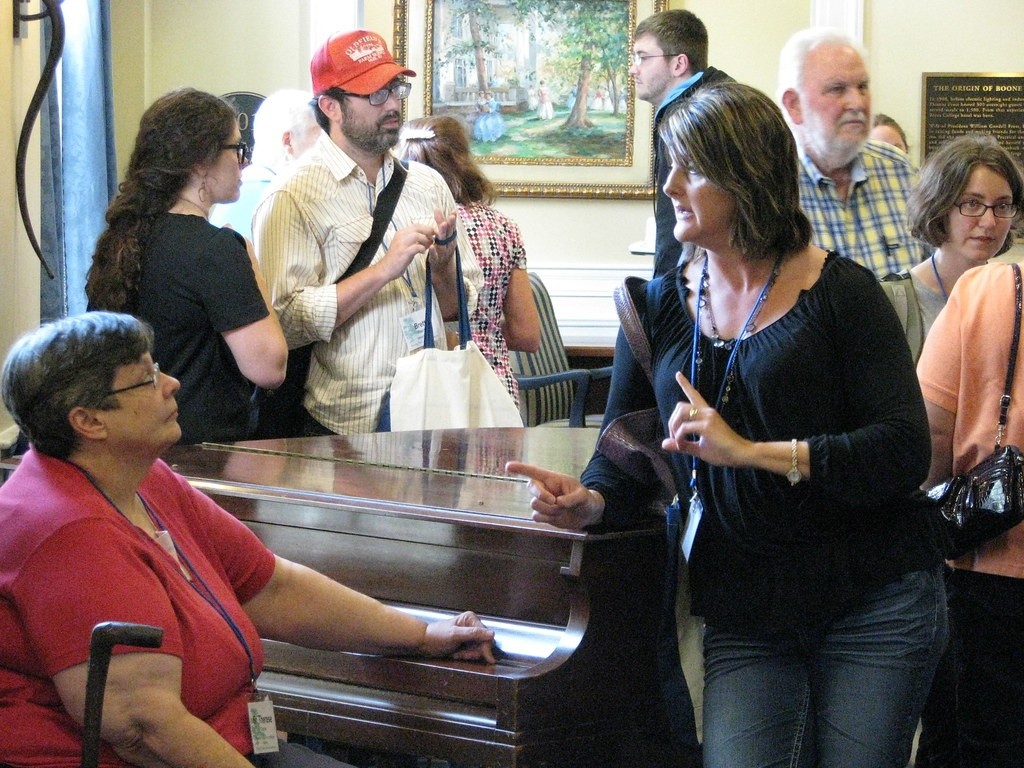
[392,0,669,201]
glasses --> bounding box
[328,82,412,106]
[953,201,1019,219]
[107,362,160,396]
[630,53,677,65]
[220,141,246,163]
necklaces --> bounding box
[696,266,781,416]
[931,253,949,300]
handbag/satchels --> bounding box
[927,446,1024,560]
[594,276,678,517]
[378,245,524,432]
[248,346,312,440]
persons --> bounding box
[251,28,483,438]
[629,10,1024,362]
[0,312,500,768]
[914,261,1024,768]
[83,89,289,448]
[206,90,322,441]
[392,115,540,412]
[505,80,950,767]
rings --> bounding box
[689,408,698,420]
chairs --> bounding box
[520,364,613,428]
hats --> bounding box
[310,30,417,95]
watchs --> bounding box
[787,439,802,486]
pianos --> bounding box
[1,423,663,768]
